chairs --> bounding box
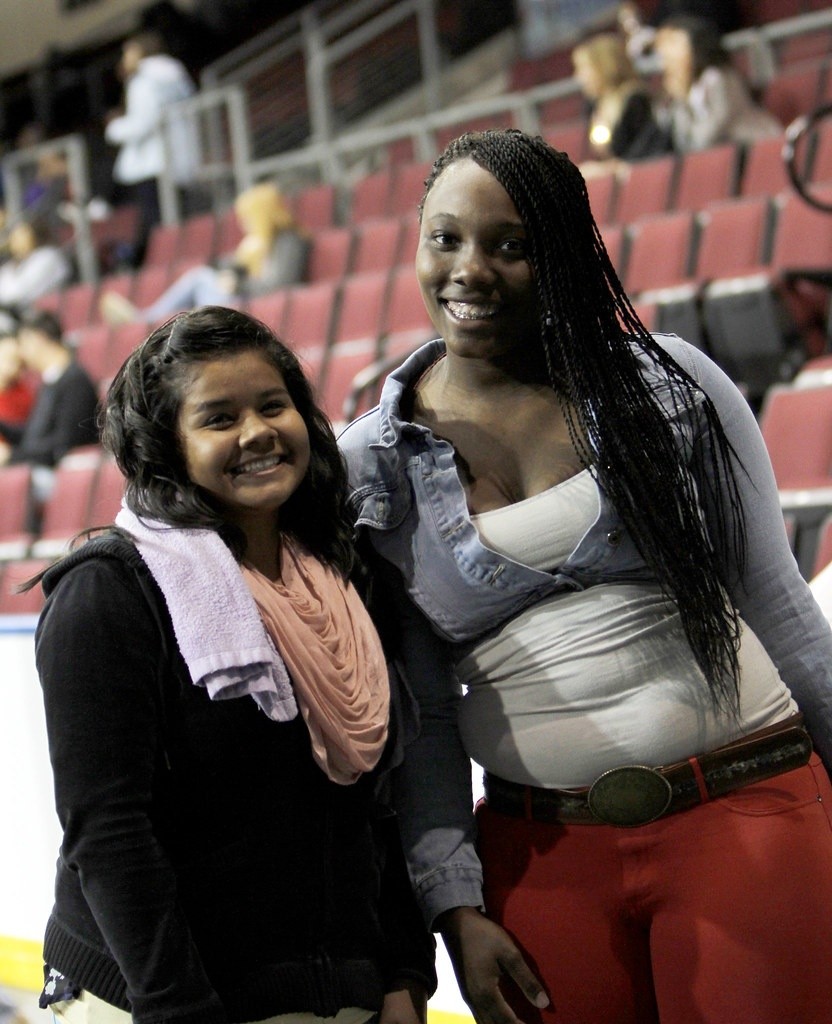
[1,3,831,615]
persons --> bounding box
[333,127,831,1023]
[35,303,437,1024]
[2,29,315,544]
[568,0,788,159]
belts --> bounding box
[484,709,812,828]
